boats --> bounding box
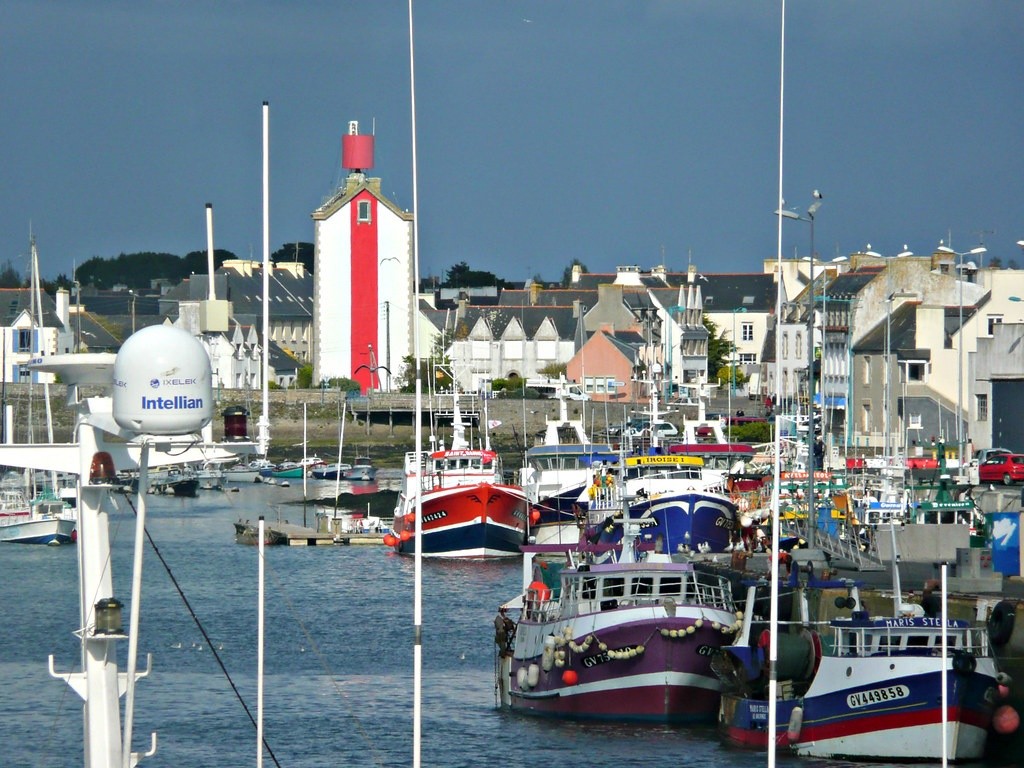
[517,372,990,553]
[492,450,749,721]
[312,456,377,480]
[202,458,259,483]
[272,463,303,478]
[199,470,227,489]
[709,513,1021,765]
[384,357,541,561]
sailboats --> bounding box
[0,234,77,548]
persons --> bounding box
[907,590,914,604]
[737,407,744,426]
[765,394,776,407]
[815,437,827,468]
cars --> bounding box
[695,427,713,436]
[623,421,679,438]
[976,448,1014,464]
[705,413,725,421]
[668,397,699,406]
[979,454,1024,486]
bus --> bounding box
[724,417,767,427]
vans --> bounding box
[526,384,593,402]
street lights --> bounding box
[668,306,685,398]
[129,289,140,333]
[774,203,823,549]
[733,307,747,396]
[803,247,987,483]
[814,295,855,447]
